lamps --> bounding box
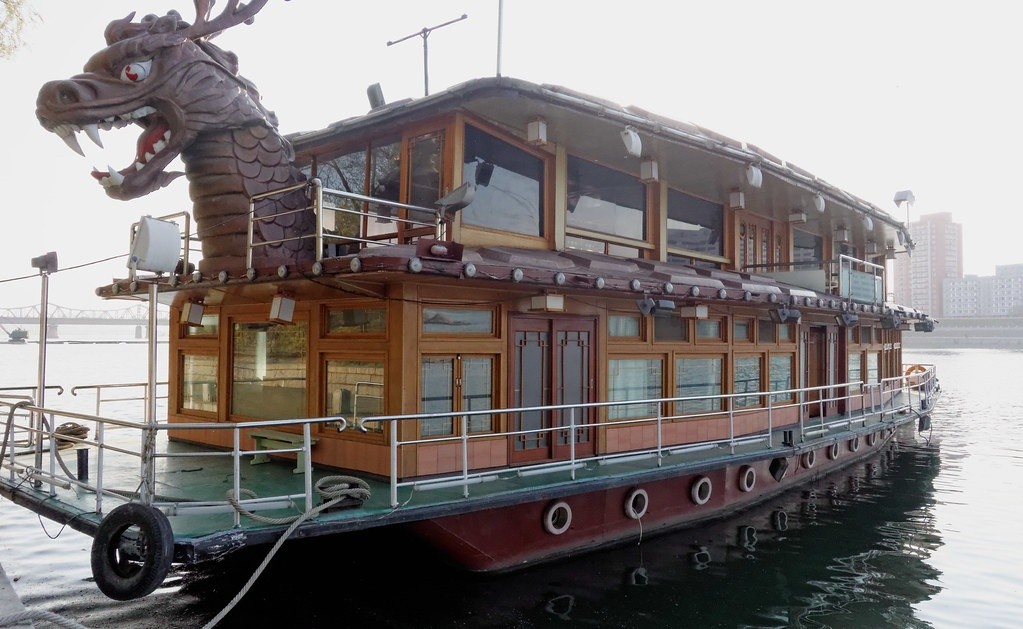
[267,292,297,326]
[619,127,641,158]
[436,182,475,210]
[905,243,912,257]
[862,214,874,230]
[835,312,934,333]
[527,116,549,147]
[885,245,897,259]
[789,210,806,224]
[638,296,709,320]
[745,161,764,188]
[180,299,206,328]
[865,237,877,254]
[639,155,659,183]
[731,188,745,210]
[770,304,801,325]
[528,290,566,313]
[813,193,826,213]
[832,224,848,243]
[896,230,905,243]
[126,218,183,288]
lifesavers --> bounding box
[91,503,175,601]
[906,365,927,386]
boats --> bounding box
[3,0,942,605]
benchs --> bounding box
[249,429,319,474]
